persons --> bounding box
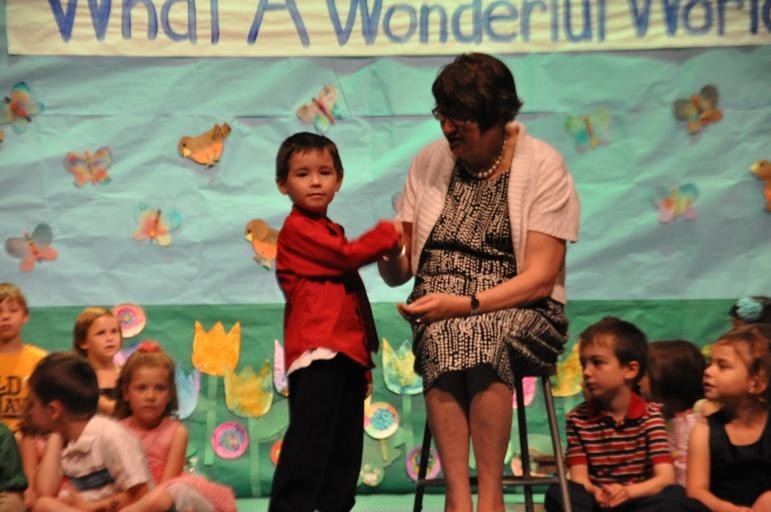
[728,294,771,334]
[375,51,580,511]
[544,316,688,511]
[686,326,771,511]
[638,335,705,492]
[0,283,237,512]
[267,127,404,509]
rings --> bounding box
[416,318,422,325]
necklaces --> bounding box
[463,131,509,179]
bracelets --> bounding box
[471,292,482,316]
[382,245,407,265]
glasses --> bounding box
[432,105,473,128]
